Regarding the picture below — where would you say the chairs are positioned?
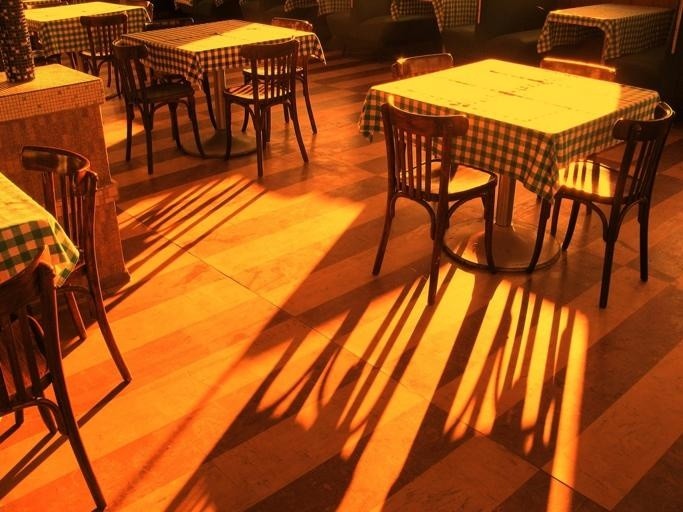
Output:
[0,141,130,433]
